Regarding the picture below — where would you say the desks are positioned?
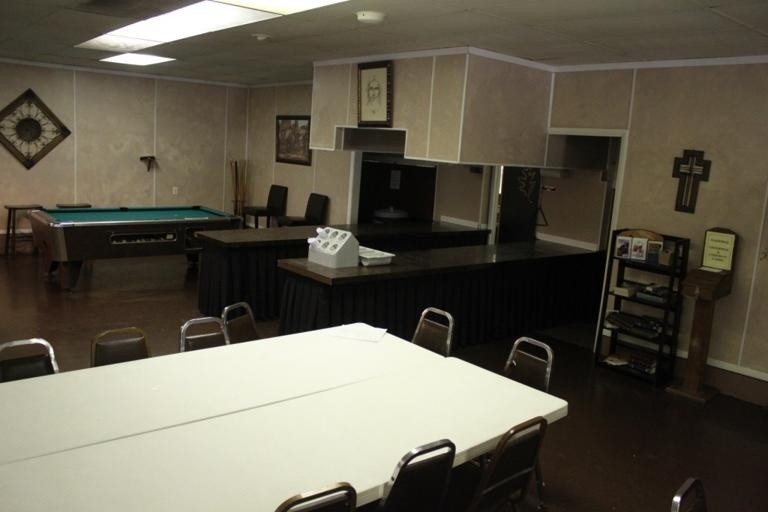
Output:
[28,206,242,290]
[195,219,492,322]
[276,240,596,334]
[0,321,569,512]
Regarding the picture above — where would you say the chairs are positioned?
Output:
[277,415,552,512]
[241,185,288,228]
[88,326,150,367]
[222,302,266,344]
[671,475,709,512]
[501,336,553,393]
[179,316,229,352]
[0,337,59,384]
[411,307,455,357]
[280,192,329,227]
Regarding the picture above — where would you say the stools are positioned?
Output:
[55,203,92,208]
[4,204,43,259]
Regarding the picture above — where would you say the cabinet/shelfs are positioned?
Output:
[585,228,691,400]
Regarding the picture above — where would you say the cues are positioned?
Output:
[229,162,246,214]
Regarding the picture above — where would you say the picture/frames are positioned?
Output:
[357,59,393,128]
[275,114,312,166]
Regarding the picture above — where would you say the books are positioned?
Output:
[630,237,649,261]
[614,236,632,260]
[614,282,677,304]
[629,356,657,374]
[646,241,664,262]
